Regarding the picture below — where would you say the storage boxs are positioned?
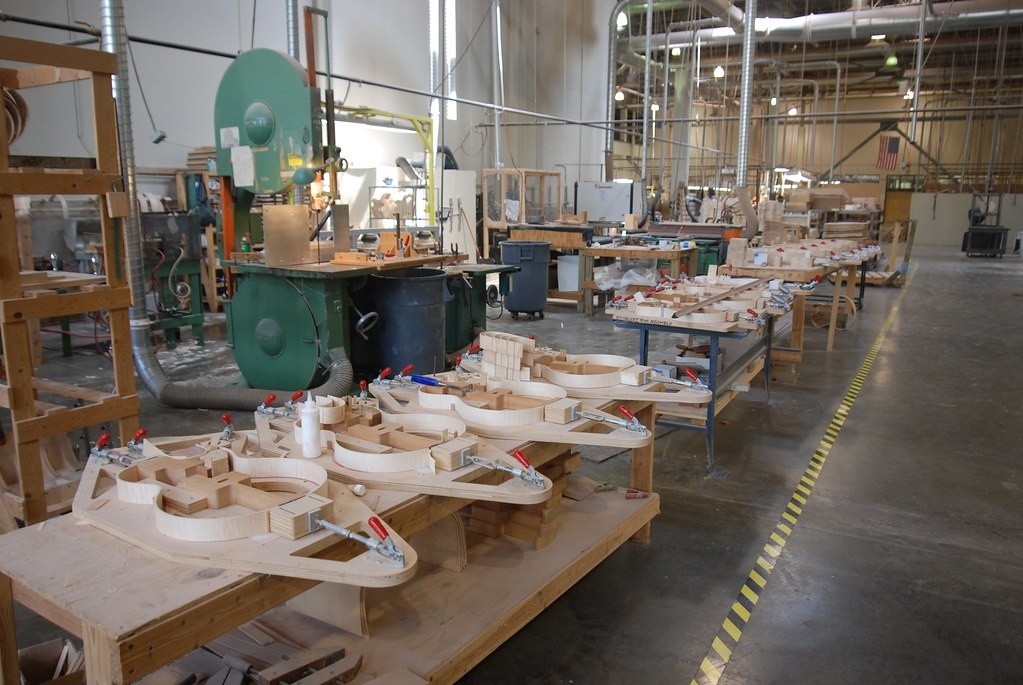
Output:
[676,350,722,373]
[654,364,679,379]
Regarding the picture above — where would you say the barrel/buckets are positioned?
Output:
[499,241,552,312]
[371,268,446,377]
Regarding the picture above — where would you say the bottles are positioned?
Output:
[301,390,322,458]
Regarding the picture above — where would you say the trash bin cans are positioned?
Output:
[367,269,452,381]
[499,240,552,321]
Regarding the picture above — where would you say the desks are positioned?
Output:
[1,362,665,684]
[582,246,699,318]
[718,242,884,379]
[605,286,799,471]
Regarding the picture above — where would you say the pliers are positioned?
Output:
[625,488,650,499]
[595,481,618,491]
